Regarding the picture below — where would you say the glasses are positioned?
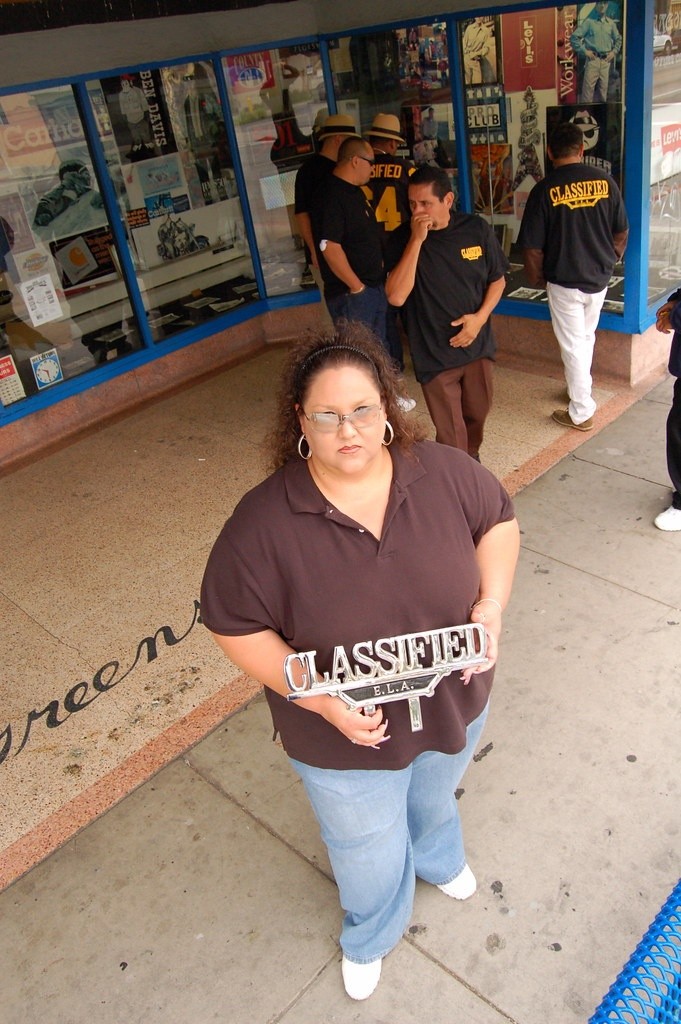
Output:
[301,403,385,433]
[357,156,375,166]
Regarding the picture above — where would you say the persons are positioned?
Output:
[655,288,681,531]
[463,18,492,84]
[570,1,622,104]
[294,113,417,412]
[31,160,132,366]
[516,123,629,432]
[201,322,519,1001]
[384,167,511,464]
[421,108,438,141]
[259,61,308,135]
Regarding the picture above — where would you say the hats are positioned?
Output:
[363,112,406,144]
[318,114,361,141]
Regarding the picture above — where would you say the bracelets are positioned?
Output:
[350,283,365,293]
[471,598,503,612]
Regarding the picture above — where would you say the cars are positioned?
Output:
[669,30,681,51]
[653,28,673,57]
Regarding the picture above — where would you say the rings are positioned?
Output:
[350,738,358,744]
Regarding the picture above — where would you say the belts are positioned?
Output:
[594,53,607,59]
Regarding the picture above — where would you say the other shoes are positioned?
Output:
[396,396,416,412]
[395,370,405,381]
[436,863,477,901]
[654,506,681,531]
[342,952,382,1000]
[553,408,593,430]
